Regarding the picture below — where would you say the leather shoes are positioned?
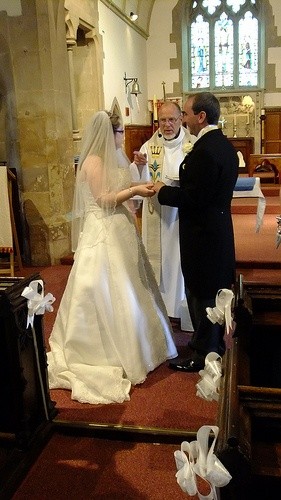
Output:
[169,356,205,371]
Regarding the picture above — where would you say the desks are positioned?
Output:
[220,274,281,500]
[249,153,281,179]
[0,272,50,500]
[225,136,254,174]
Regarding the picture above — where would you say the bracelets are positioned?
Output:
[129,188,134,198]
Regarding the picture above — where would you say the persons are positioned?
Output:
[129,102,196,333]
[45,109,179,405]
[148,92,240,374]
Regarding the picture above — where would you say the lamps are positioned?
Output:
[130,12,139,21]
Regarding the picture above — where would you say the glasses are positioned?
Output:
[158,115,182,125]
[114,129,124,135]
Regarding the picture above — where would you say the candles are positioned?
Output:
[233,113,236,125]
[247,110,250,124]
